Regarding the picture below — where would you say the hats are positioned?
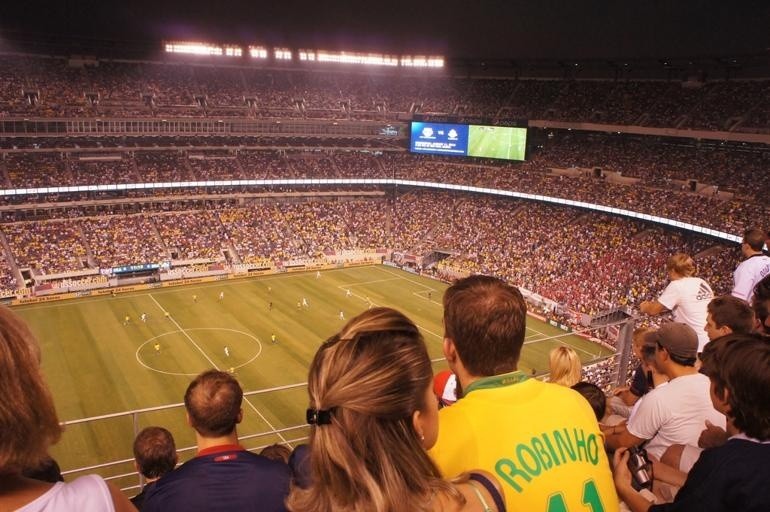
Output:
[644,322,698,359]
[432,371,457,406]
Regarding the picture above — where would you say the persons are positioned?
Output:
[0,47,769,512]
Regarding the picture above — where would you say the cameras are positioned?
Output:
[620,444,653,488]
